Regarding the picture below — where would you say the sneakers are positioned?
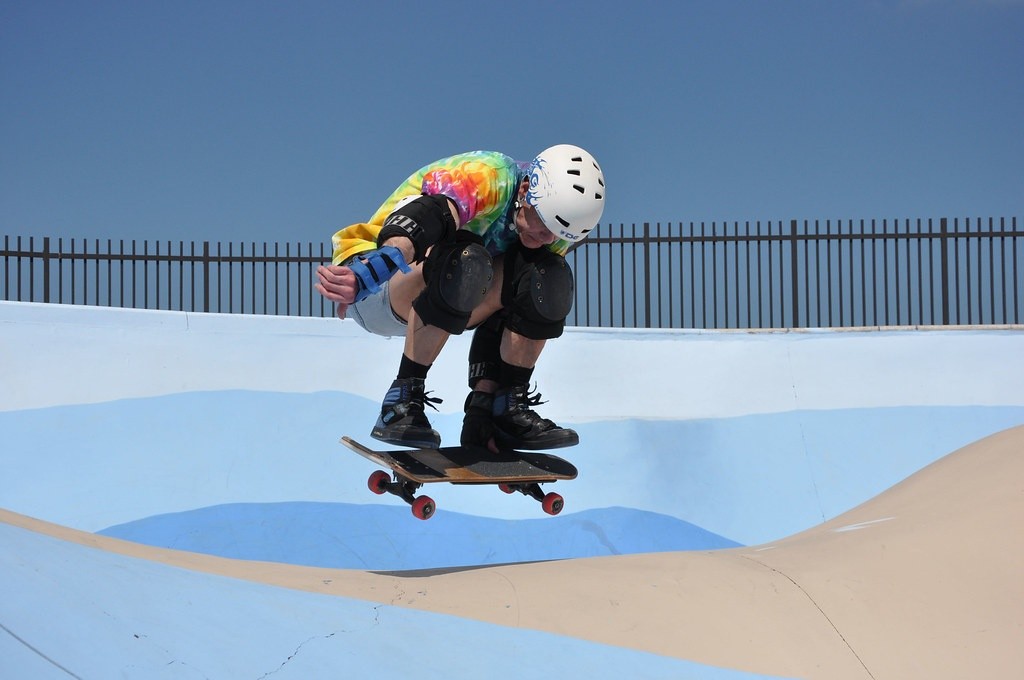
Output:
[370,378,443,451]
[461,391,580,451]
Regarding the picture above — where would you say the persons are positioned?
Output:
[315,144,605,453]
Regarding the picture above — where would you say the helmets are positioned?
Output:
[526,143,606,242]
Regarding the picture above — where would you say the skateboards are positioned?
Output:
[339,434,580,521]
